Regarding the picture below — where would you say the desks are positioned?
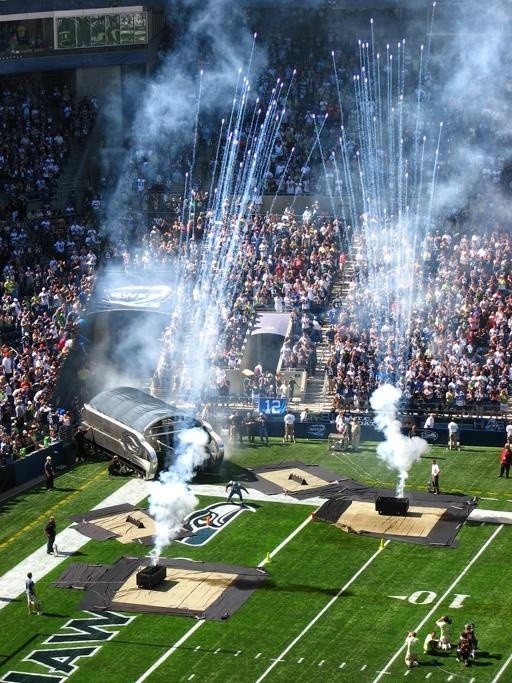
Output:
[327,433,351,452]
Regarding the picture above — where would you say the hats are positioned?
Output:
[46,456,52,460]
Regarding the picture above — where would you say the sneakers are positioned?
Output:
[424,651,430,655]
[444,642,475,667]
[410,661,421,667]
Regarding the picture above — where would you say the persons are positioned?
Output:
[403,631,419,667]
[498,442,512,478]
[45,456,58,491]
[422,630,442,654]
[434,615,453,650]
[455,632,471,666]
[0,1,511,468]
[43,515,57,555]
[106,454,122,476]
[427,458,441,494]
[462,623,477,660]
[25,572,44,615]
[224,480,250,503]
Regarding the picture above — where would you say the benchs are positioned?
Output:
[408,429,438,446]
